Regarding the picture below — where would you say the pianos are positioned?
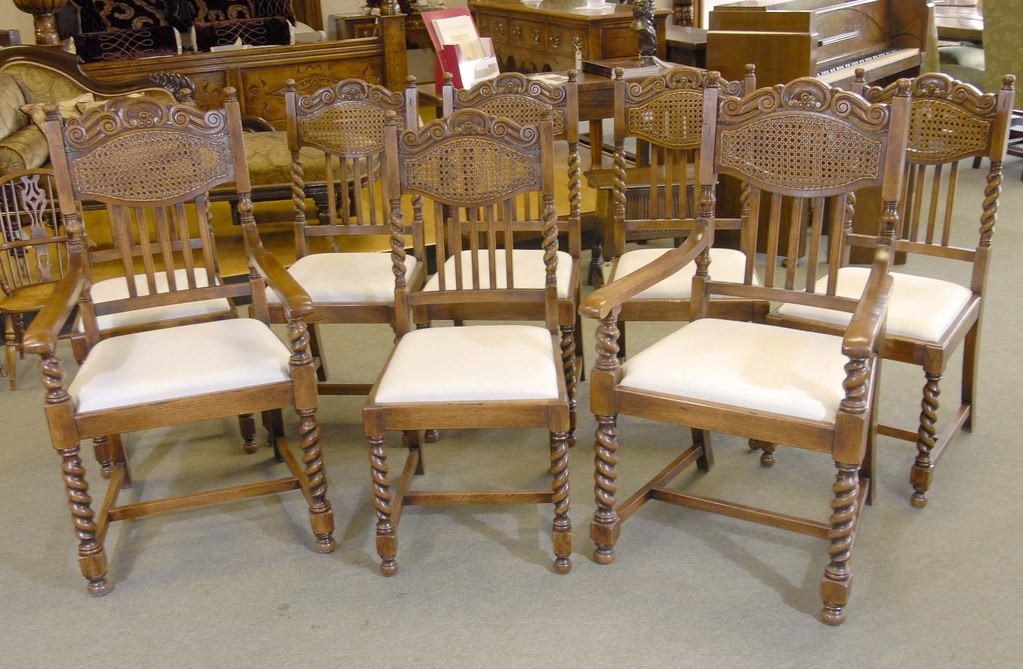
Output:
[705,0,935,266]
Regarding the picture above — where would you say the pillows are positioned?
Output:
[75,91,147,115]
[18,92,98,143]
[0,125,51,184]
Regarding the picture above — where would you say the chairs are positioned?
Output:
[242,78,434,452]
[753,69,1017,508]
[577,69,915,630]
[362,101,580,574]
[71,186,257,479]
[21,86,336,598]
[594,63,775,451]
[412,68,584,449]
[0,168,82,391]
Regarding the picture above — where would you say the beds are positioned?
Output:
[0,53,381,231]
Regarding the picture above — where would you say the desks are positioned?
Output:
[465,1,674,77]
[331,9,439,51]
